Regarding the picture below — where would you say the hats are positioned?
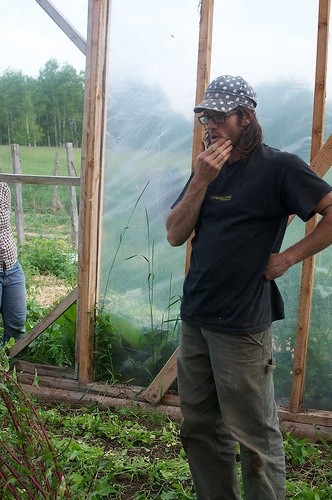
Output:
[193,75,258,114]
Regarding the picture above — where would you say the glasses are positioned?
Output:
[198,112,239,125]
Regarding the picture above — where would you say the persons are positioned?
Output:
[166,74,332,500]
[1,182,27,358]
